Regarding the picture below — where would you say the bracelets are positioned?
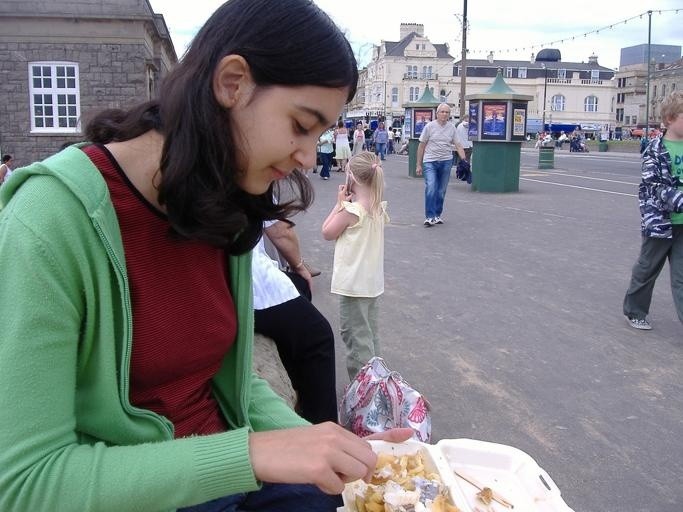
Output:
[295,258,307,271]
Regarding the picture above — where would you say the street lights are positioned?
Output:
[539,63,548,133]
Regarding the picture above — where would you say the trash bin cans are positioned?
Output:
[539,145,554,168]
[409,137,424,178]
[597,139,608,152]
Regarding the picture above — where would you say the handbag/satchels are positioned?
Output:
[337,356,435,445]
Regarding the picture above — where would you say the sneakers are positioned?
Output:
[623,311,652,331]
[285,257,322,277]
[423,218,435,226]
[323,158,386,181]
[434,216,444,224]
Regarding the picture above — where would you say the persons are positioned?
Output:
[619,90,682,331]
[415,102,473,229]
[313,113,400,180]
[0,3,428,512]
[0,153,13,184]
[532,129,665,153]
[240,195,335,434]
[317,148,389,394]
